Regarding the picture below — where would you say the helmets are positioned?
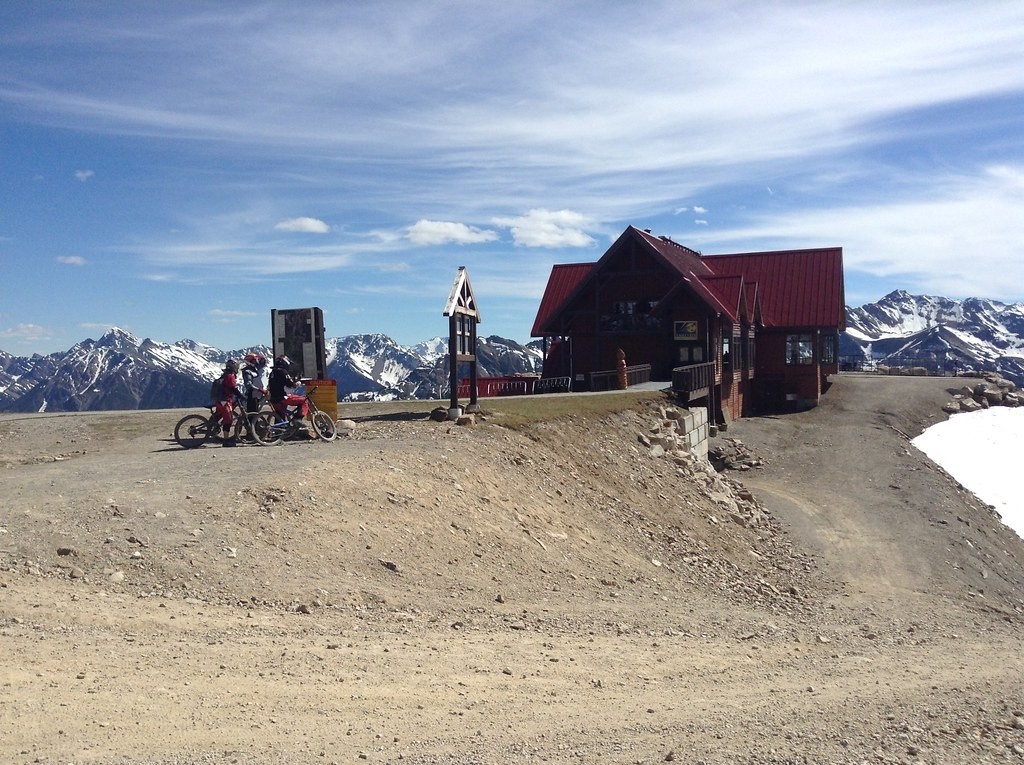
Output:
[225,358,240,374]
[275,355,295,367]
[243,354,259,368]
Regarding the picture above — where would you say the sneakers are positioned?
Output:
[295,420,308,428]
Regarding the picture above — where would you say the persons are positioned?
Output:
[209,359,247,447]
[268,356,308,440]
[241,353,269,440]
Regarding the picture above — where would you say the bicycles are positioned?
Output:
[250,385,336,447]
[235,388,301,445]
[174,386,269,448]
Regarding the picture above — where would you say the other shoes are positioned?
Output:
[222,442,237,447]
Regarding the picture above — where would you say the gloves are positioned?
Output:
[259,388,269,394]
[295,380,302,387]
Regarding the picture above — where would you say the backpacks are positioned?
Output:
[210,374,227,401]
